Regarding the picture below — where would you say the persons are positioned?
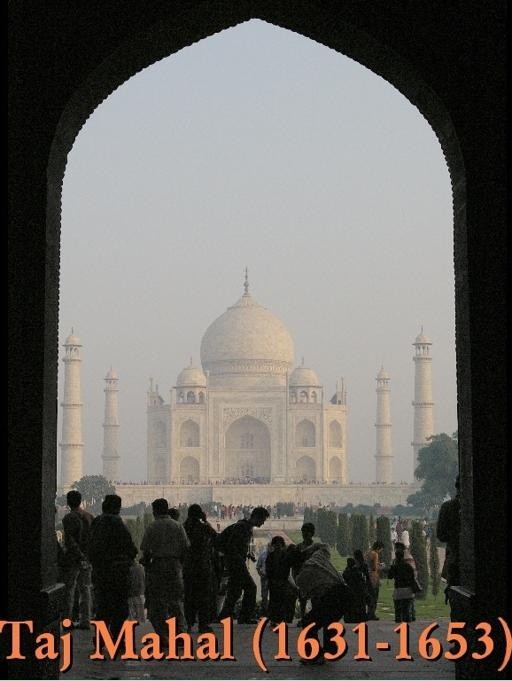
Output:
[54,490,433,665]
[436,474,460,657]
